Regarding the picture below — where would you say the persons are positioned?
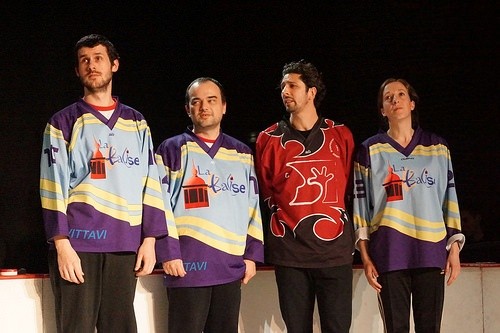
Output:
[354,79,465,333]
[154,77,264,333]
[255,63,354,333]
[40,33,169,333]
[460,206,500,263]
